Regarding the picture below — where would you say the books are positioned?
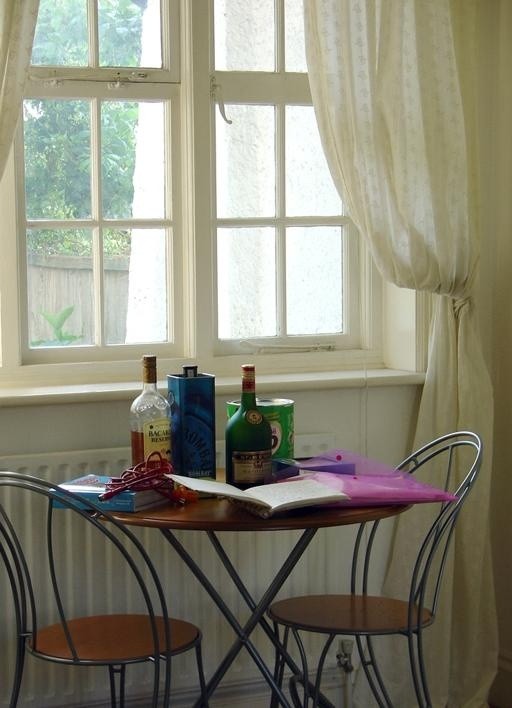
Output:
[48,473,171,513]
[163,471,352,519]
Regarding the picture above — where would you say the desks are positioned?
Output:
[90,466,412,707]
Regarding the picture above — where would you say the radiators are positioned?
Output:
[1,433,354,707]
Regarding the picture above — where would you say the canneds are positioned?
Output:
[226,398,293,462]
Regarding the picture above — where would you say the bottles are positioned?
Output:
[128,355,173,472]
[225,364,271,491]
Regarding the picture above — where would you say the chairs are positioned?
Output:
[0,470,207,708]
[269,431,483,707]
